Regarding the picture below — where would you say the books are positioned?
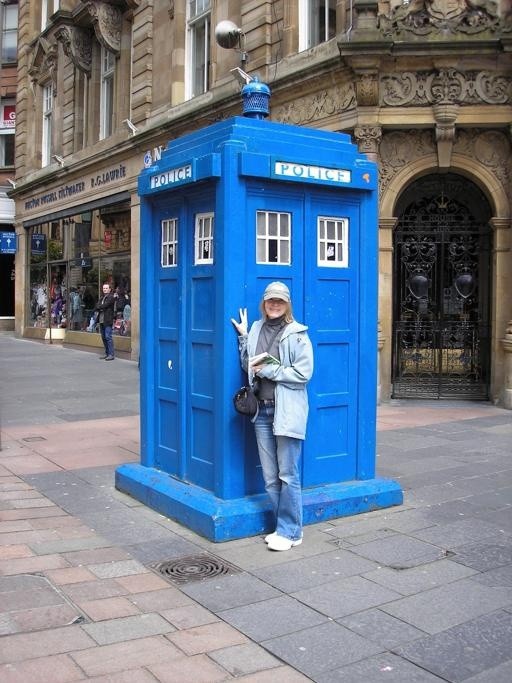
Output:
[249,351,280,366]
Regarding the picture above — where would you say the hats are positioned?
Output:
[264,281,290,303]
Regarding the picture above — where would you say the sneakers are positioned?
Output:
[99,355,114,360]
[264,530,304,551]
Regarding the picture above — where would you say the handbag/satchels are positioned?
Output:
[233,386,257,416]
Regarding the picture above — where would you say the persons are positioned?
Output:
[229,279,316,552]
[94,281,118,360]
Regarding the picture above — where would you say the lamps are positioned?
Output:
[214,19,248,60]
